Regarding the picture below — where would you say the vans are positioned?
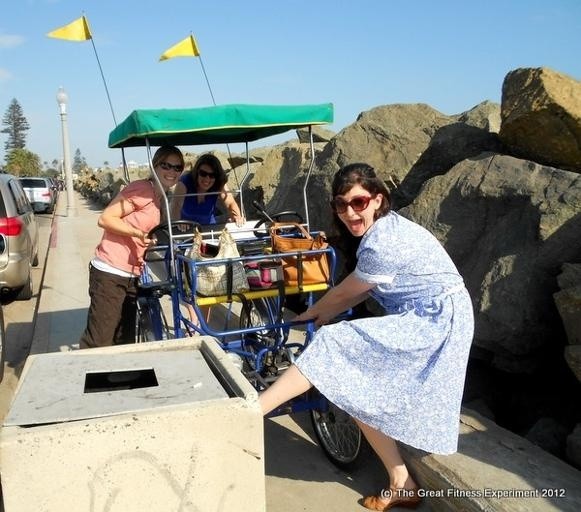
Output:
[0,173,40,302]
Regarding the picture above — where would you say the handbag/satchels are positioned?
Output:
[270,223,330,286]
[184,225,250,295]
[244,259,285,291]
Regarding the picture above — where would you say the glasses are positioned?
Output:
[158,161,182,172]
[198,168,217,179]
[329,195,376,214]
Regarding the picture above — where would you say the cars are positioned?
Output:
[17,175,57,214]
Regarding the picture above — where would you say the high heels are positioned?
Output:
[362,488,422,511]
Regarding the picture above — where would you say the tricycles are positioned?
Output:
[105,100,369,469]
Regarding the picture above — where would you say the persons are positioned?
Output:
[171,153,248,232]
[78,146,183,348]
[256,162,477,512]
